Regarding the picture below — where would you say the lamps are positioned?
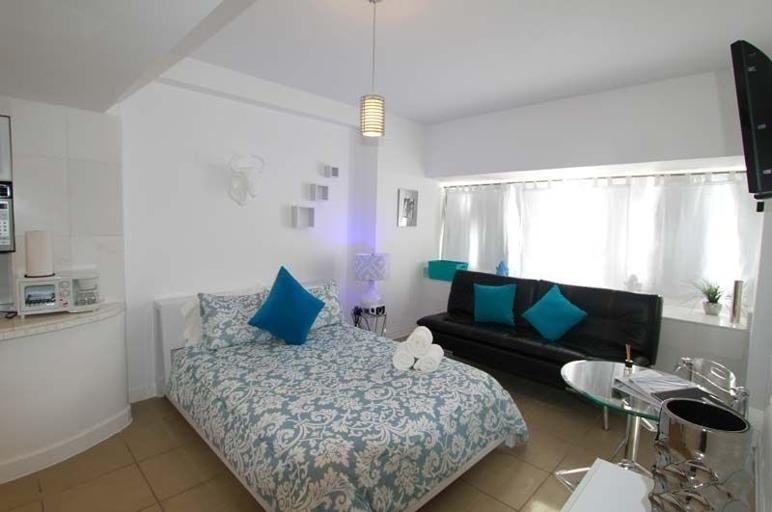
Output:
[353,251,390,306]
[361,0,385,136]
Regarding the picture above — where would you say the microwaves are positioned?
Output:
[16,270,73,320]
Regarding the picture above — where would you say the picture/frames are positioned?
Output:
[398,189,418,228]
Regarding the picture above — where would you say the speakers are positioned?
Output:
[370,303,386,316]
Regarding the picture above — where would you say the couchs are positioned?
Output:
[417,269,663,431]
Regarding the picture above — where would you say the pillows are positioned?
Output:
[198,266,345,350]
[473,283,517,325]
[521,284,588,343]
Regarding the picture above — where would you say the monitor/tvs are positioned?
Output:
[730,37,772,212]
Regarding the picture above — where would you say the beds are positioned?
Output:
[154,285,529,512]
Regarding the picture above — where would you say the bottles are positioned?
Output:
[623,358,634,376]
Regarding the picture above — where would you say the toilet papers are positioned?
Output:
[24,229,54,277]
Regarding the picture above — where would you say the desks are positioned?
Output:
[561,359,719,479]
[554,455,654,511]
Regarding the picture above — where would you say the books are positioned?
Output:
[651,386,725,406]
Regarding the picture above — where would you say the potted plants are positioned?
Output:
[679,278,733,316]
